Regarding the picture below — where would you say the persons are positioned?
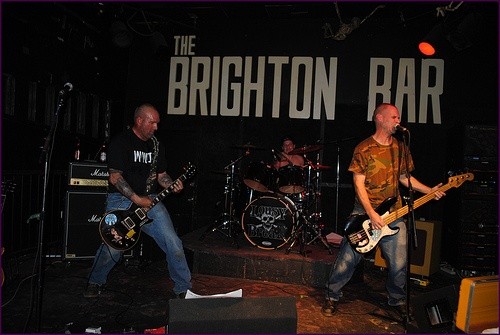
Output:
[322,103,446,324]
[84,104,193,300]
[275,138,302,187]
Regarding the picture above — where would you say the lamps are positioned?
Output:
[418,37,440,56]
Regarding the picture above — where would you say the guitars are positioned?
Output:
[99,162,197,250]
[344,173,474,254]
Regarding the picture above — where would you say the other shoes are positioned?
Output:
[179,292,187,299]
[396,303,408,316]
[321,299,336,317]
[84,283,99,297]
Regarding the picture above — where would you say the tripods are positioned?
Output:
[203,149,340,257]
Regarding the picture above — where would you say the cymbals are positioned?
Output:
[230,145,267,152]
[289,145,322,155]
[301,164,334,168]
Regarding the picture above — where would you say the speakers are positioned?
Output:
[64,191,111,260]
[374,220,441,276]
[412,283,460,335]
[167,296,298,335]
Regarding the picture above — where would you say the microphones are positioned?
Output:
[59,83,73,95]
[394,123,408,132]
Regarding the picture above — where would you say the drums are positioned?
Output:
[276,164,303,193]
[242,193,300,250]
[242,161,278,192]
